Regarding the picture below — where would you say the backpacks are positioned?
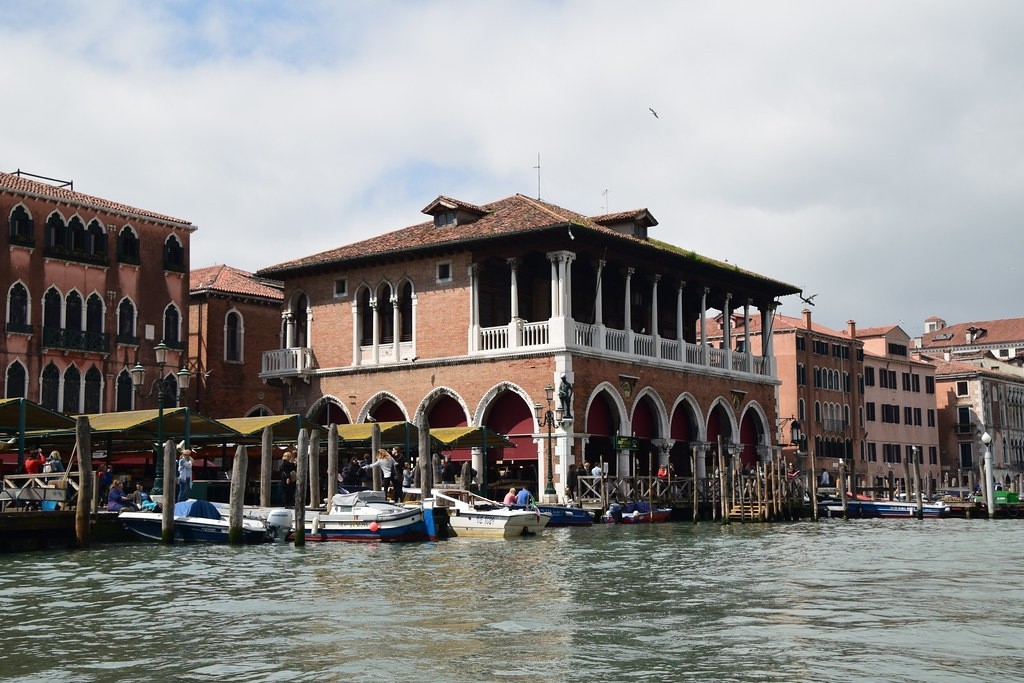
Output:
[42,459,56,473]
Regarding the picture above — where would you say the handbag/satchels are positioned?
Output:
[14,463,25,488]
[281,475,291,487]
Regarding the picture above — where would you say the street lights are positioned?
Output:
[534,383,564,494]
[130,340,192,494]
[982,432,995,514]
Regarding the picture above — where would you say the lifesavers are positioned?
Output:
[1006,504,1020,518]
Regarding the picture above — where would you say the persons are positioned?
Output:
[441,455,456,483]
[504,486,531,505]
[558,374,573,415]
[821,468,830,487]
[98,464,113,508]
[659,463,675,495]
[134,482,158,512]
[402,456,421,501]
[346,453,373,486]
[26,448,46,474]
[44,450,65,482]
[177,450,193,502]
[108,479,138,512]
[788,462,800,477]
[280,450,297,508]
[742,464,756,475]
[567,462,601,502]
[362,445,405,503]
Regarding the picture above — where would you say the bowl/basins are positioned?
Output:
[41,501,58,511]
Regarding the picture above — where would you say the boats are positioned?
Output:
[286,491,423,542]
[117,491,292,544]
[602,503,672,524]
[802,482,1024,518]
[537,505,596,527]
[398,488,553,538]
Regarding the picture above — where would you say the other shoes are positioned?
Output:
[285,505,293,509]
